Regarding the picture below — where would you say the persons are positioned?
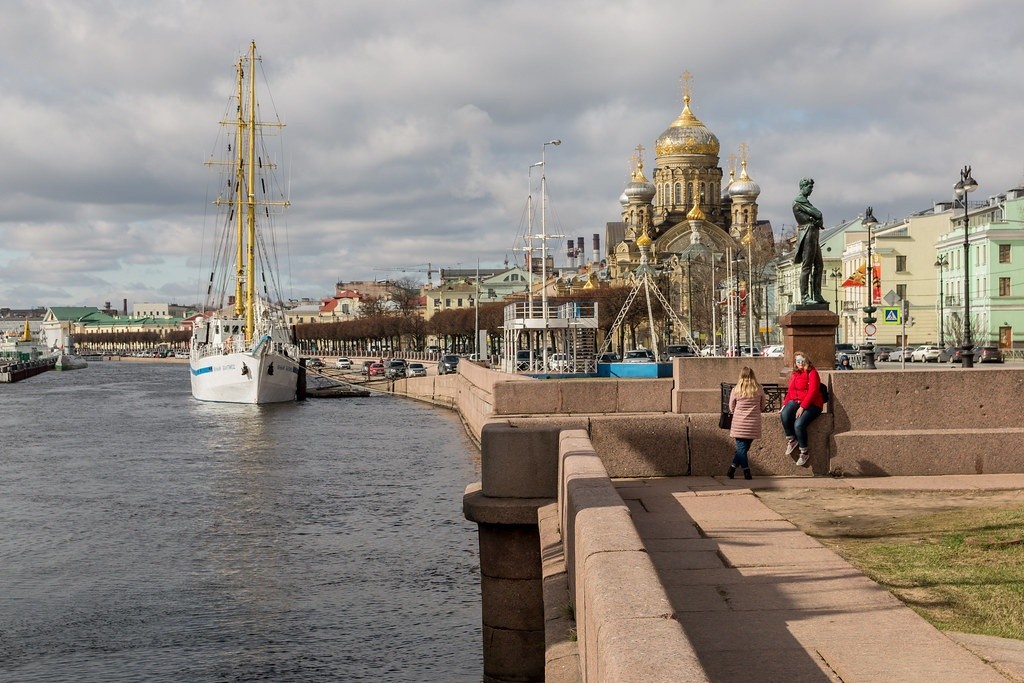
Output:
[792,176,830,305]
[836,355,854,370]
[729,366,766,479]
[779,351,823,466]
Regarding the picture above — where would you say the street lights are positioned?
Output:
[526,139,562,372]
[952,164,978,369]
[829,266,843,344]
[860,206,878,369]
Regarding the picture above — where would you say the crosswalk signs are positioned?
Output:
[885,310,898,321]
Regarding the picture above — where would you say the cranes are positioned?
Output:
[373,261,440,283]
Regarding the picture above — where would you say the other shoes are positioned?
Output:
[727,466,736,479]
[785,440,798,455]
[796,454,809,466]
[744,469,752,479]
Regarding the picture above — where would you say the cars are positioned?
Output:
[119,350,190,359]
[336,358,351,370]
[423,346,440,354]
[437,355,462,375]
[361,360,384,376]
[836,343,1004,364]
[517,349,541,369]
[89,350,114,356]
[302,357,322,366]
[549,353,574,370]
[595,343,786,363]
[385,358,428,379]
[469,354,490,363]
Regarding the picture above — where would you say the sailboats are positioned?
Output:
[188,39,306,404]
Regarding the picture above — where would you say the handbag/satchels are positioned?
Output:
[820,382,828,404]
[719,411,733,429]
